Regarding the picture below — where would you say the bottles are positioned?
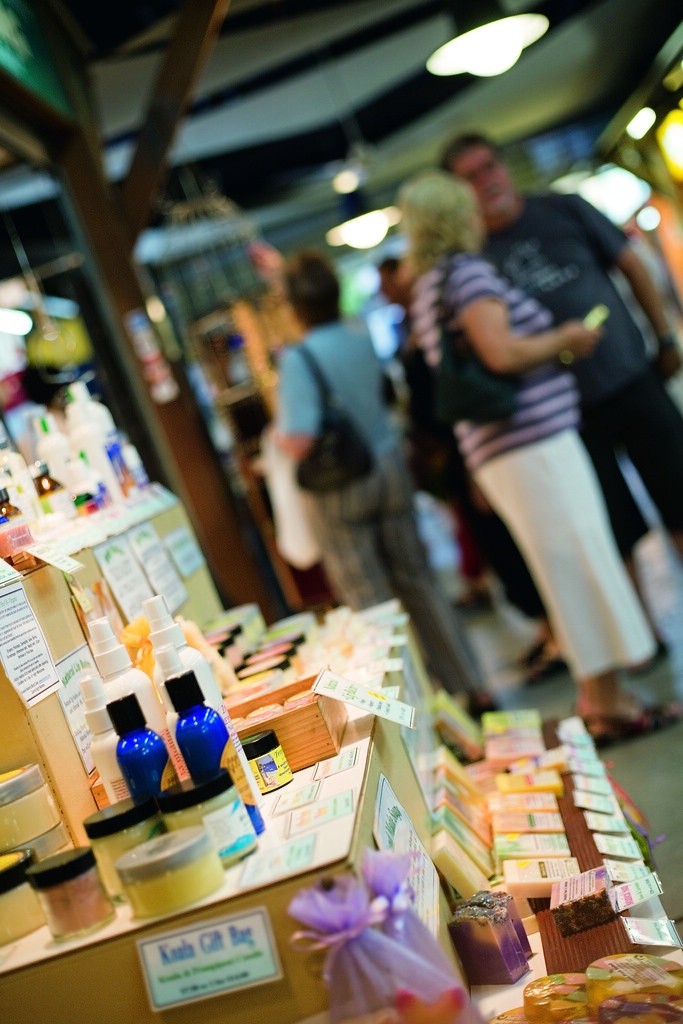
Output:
[142,594,265,810]
[153,643,182,757]
[106,692,180,799]
[165,669,266,835]
[0,381,147,556]
[87,616,191,783]
[76,674,132,805]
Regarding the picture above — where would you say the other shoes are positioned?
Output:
[593,700,681,750]
[466,690,498,721]
[523,641,545,666]
[453,590,491,610]
[631,641,667,674]
[526,658,566,684]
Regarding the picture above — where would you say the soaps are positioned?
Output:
[447,890,533,984]
[550,866,618,937]
[429,688,582,902]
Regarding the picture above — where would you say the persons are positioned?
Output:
[399,171,682,747]
[440,132,683,679]
[377,257,567,691]
[274,250,502,722]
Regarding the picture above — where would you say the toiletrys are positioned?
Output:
[0,380,149,558]
[142,595,266,813]
[165,671,266,836]
[106,693,180,798]
[87,616,191,783]
[80,674,131,805]
[154,644,218,778]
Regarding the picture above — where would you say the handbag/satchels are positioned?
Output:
[260,432,322,569]
[432,256,518,423]
[295,345,377,492]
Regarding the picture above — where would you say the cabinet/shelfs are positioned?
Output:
[0,502,683,1023]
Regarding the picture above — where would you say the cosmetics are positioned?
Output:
[1,382,370,937]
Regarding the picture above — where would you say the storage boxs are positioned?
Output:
[228,675,349,772]
